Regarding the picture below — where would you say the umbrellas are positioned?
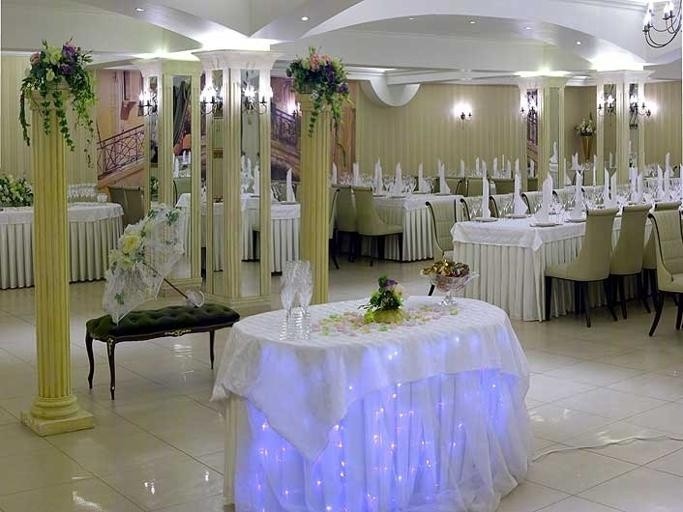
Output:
[101,208,206,322]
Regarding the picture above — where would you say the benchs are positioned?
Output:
[85,303,240,400]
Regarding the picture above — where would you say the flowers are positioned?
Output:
[281,44,356,168]
[0,169,34,210]
[18,36,98,168]
[574,117,598,137]
[356,273,408,325]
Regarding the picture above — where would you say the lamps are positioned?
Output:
[595,91,654,129]
[196,85,224,118]
[240,83,274,115]
[642,0,681,48]
[137,90,158,117]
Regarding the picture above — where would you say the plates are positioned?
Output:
[529,221,563,228]
[436,193,450,196]
[565,217,586,223]
[373,194,386,197]
[413,191,427,195]
[250,194,260,198]
[281,200,297,205]
[505,214,527,218]
[472,216,499,222]
[391,194,406,198]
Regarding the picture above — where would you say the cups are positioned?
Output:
[281,260,296,325]
[297,260,311,325]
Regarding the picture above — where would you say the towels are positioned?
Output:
[610,152,613,168]
[183,151,186,162]
[571,155,578,170]
[506,160,511,179]
[679,164,683,199]
[173,152,175,168]
[631,167,636,205]
[419,163,423,190]
[286,167,295,201]
[460,159,465,177]
[254,165,259,195]
[241,154,245,171]
[665,152,674,177]
[661,170,670,203]
[438,158,441,174]
[482,160,487,178]
[482,177,491,219]
[531,174,552,222]
[247,157,251,177]
[529,159,535,179]
[611,171,617,210]
[551,142,558,163]
[439,163,450,193]
[332,161,337,184]
[514,174,528,215]
[574,152,578,165]
[515,157,520,175]
[188,151,191,164]
[493,156,497,175]
[353,162,360,185]
[593,154,597,169]
[476,157,479,175]
[174,158,180,178]
[604,168,611,209]
[375,159,383,195]
[628,140,632,156]
[570,170,584,219]
[637,171,644,204]
[395,162,402,196]
[658,166,662,198]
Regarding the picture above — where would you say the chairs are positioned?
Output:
[434,179,462,195]
[464,177,491,197]
[610,203,652,321]
[643,200,683,337]
[543,207,619,328]
[316,186,342,274]
[352,186,406,269]
[424,181,683,298]
[491,178,538,194]
[336,186,360,263]
